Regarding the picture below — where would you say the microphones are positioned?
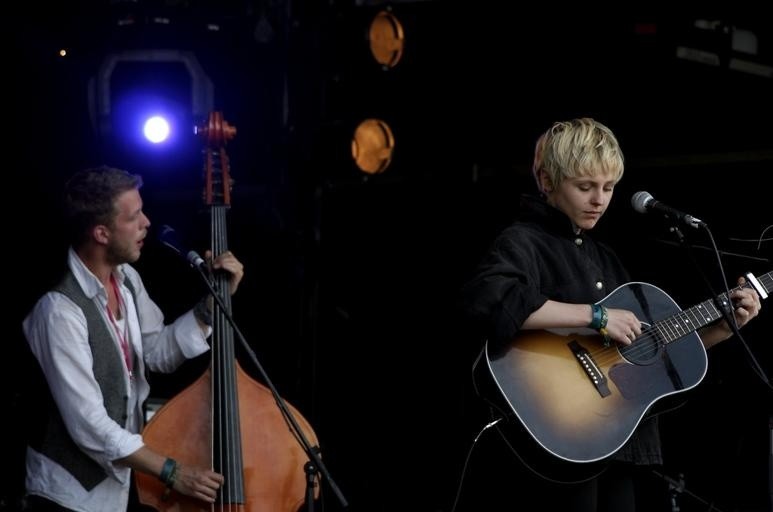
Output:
[154,223,205,267]
[631,189,704,230]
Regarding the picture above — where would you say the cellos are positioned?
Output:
[129,113,321,510]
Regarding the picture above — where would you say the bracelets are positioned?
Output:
[588,303,603,331]
[159,457,176,484]
[194,297,213,327]
[595,304,608,335]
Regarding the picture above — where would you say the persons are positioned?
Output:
[453,112,763,511]
[13,163,247,511]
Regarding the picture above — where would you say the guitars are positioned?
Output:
[470,270,773,485]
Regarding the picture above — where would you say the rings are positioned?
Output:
[628,331,634,338]
